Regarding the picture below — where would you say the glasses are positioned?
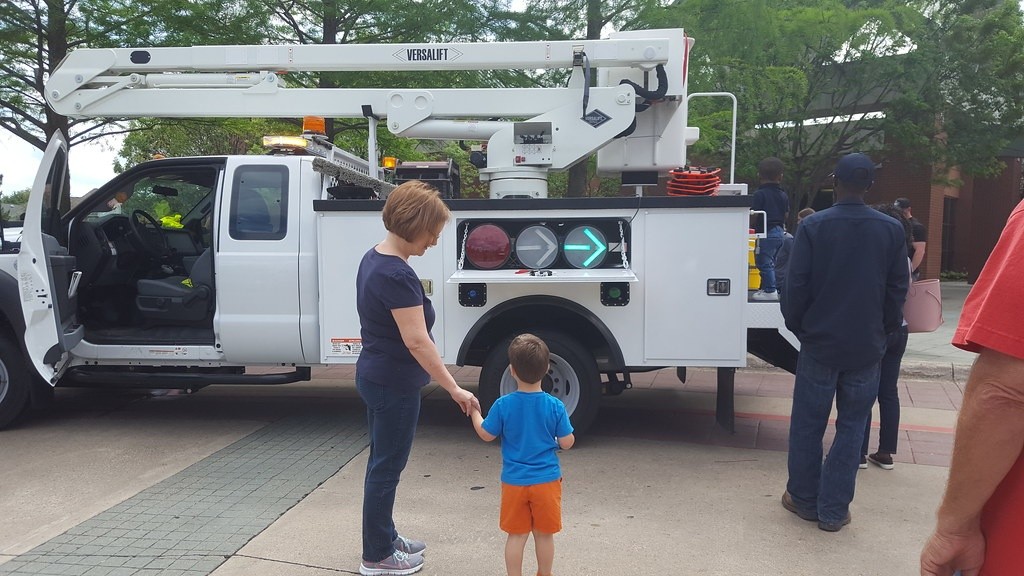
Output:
[427,228,440,242]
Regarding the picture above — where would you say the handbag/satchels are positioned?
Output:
[900,257,944,332]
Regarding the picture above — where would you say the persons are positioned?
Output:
[471,333,575,576]
[748,157,788,301]
[921,199,1024,576]
[796,208,815,226]
[782,154,909,531]
[858,198,927,469]
[356,181,482,576]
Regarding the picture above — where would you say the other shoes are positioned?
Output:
[782,490,818,520]
[817,510,851,531]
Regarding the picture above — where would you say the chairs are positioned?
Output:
[134,189,273,326]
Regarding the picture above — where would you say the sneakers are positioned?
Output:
[859,455,867,468]
[868,452,894,469]
[391,535,427,556]
[753,289,778,299]
[358,549,423,576]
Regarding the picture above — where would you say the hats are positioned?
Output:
[892,197,912,209]
[757,157,785,180]
[828,152,876,182]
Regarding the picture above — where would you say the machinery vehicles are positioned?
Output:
[0,28,801,430]
